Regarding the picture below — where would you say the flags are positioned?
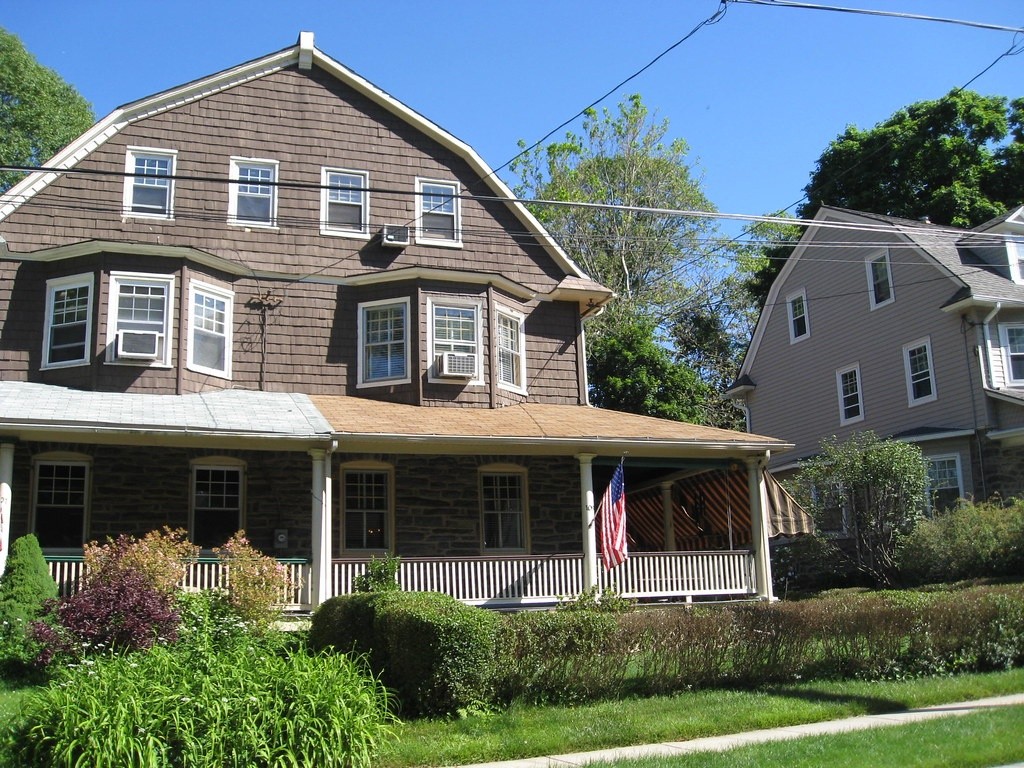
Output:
[600,457,628,571]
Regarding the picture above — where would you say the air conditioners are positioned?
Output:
[381,224,410,247]
[118,329,159,360]
[437,350,478,378]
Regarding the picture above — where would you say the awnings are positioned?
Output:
[625,465,814,553]
